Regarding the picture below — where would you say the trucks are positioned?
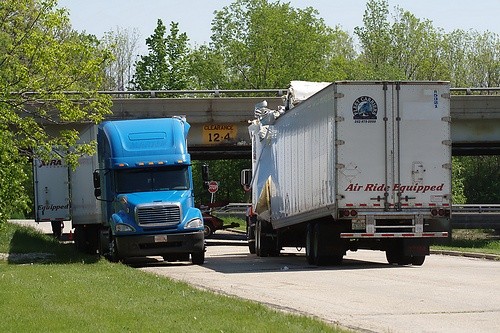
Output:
[32,116,205,266]
[240,81,453,264]
